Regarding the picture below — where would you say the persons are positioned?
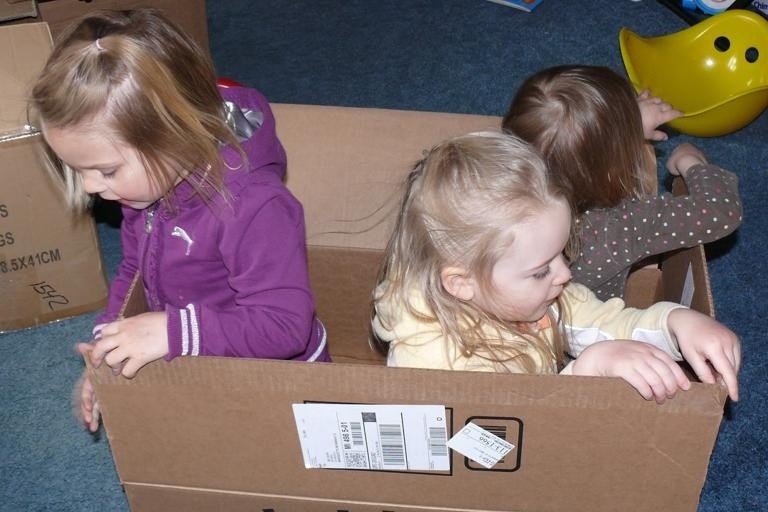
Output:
[367,130,742,408]
[26,7,334,433]
[498,64,742,304]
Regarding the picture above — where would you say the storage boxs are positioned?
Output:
[81,103,728,512]
